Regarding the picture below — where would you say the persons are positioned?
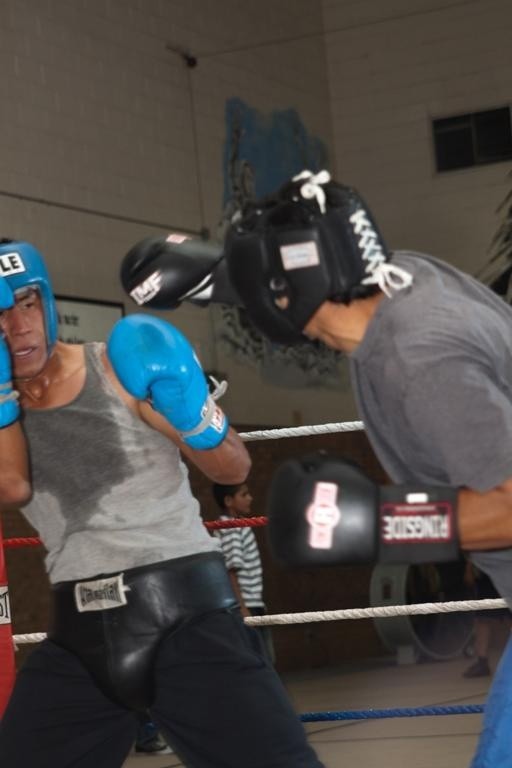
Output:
[213,481,276,666]
[121,173,512,768]
[462,564,507,679]
[136,721,173,754]
[0,235,324,768]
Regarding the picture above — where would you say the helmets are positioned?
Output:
[224,171,390,343]
[0,241,57,354]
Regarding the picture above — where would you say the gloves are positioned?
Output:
[120,235,224,308]
[0,274,21,428]
[270,451,378,570]
[108,313,228,450]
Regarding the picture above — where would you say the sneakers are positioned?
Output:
[136,741,173,754]
[463,662,489,676]
